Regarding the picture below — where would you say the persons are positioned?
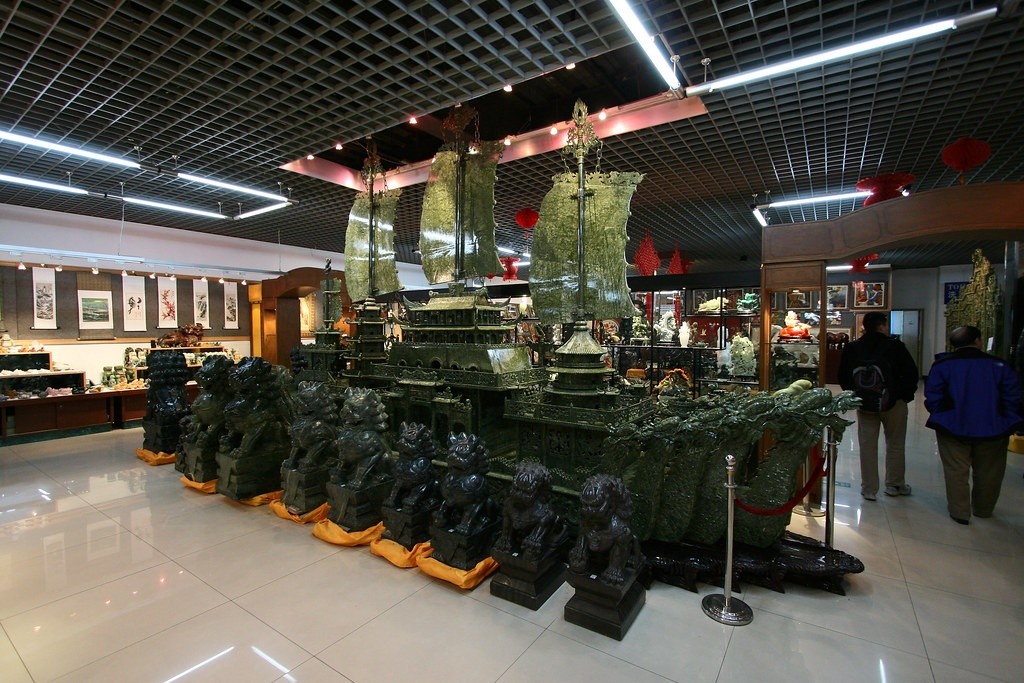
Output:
[838,312,919,502]
[923,325,1024,525]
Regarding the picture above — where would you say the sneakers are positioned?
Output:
[884,484,911,496]
[861,489,876,500]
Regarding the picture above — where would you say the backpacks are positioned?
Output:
[849,363,889,411]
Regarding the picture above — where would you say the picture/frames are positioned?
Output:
[723,289,745,312]
[749,286,778,311]
[852,280,886,309]
[692,289,716,311]
[818,285,848,310]
[30,265,240,341]
[783,291,812,310]
